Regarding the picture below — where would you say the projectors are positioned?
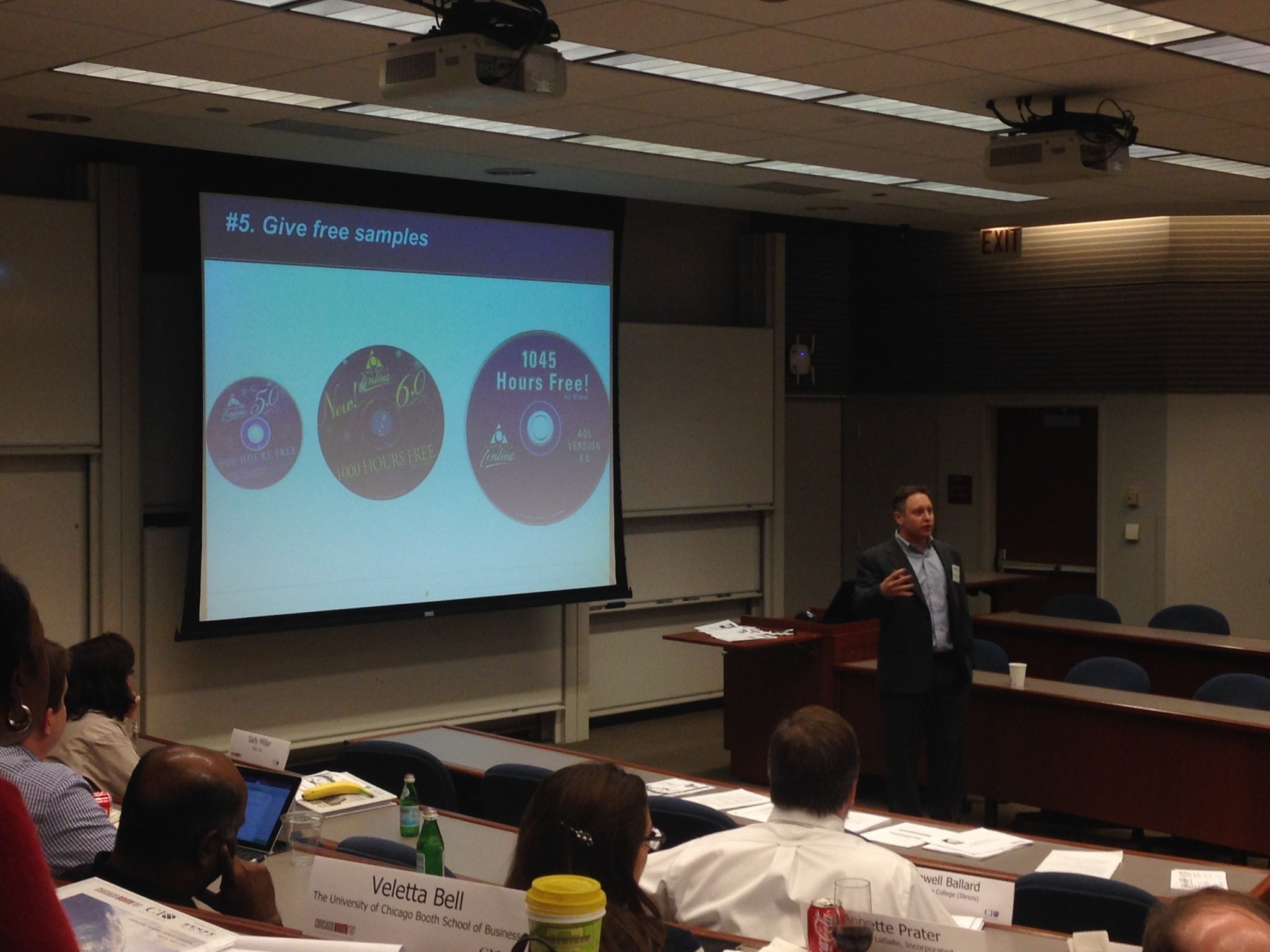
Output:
[377,31,570,104]
[983,128,1130,184]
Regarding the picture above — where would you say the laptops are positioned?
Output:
[235,763,304,866]
[815,578,854,624]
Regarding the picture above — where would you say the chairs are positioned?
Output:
[325,594,1270,952]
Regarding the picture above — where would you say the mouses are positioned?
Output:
[794,610,815,620]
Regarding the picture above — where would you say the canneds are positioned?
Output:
[94,791,113,818]
[807,898,845,952]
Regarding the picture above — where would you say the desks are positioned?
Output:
[957,607,1270,664]
[341,723,1270,932]
[113,771,1142,952]
[840,653,1270,861]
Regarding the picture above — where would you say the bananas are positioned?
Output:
[301,781,374,800]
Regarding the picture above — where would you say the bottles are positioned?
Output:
[399,773,421,839]
[415,806,445,878]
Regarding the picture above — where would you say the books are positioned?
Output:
[846,810,957,849]
[55,876,239,952]
[923,827,1036,861]
[952,914,985,931]
[295,770,397,819]
[646,776,775,825]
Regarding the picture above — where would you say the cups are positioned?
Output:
[523,875,607,952]
[279,811,326,867]
[830,878,874,952]
[1009,663,1027,687]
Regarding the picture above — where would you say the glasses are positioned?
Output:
[634,827,665,850]
[126,667,135,677]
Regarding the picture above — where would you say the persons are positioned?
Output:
[43,632,141,805]
[850,485,975,824]
[637,705,958,950]
[0,639,120,880]
[59,745,284,927]
[503,762,703,952]
[0,566,79,952]
